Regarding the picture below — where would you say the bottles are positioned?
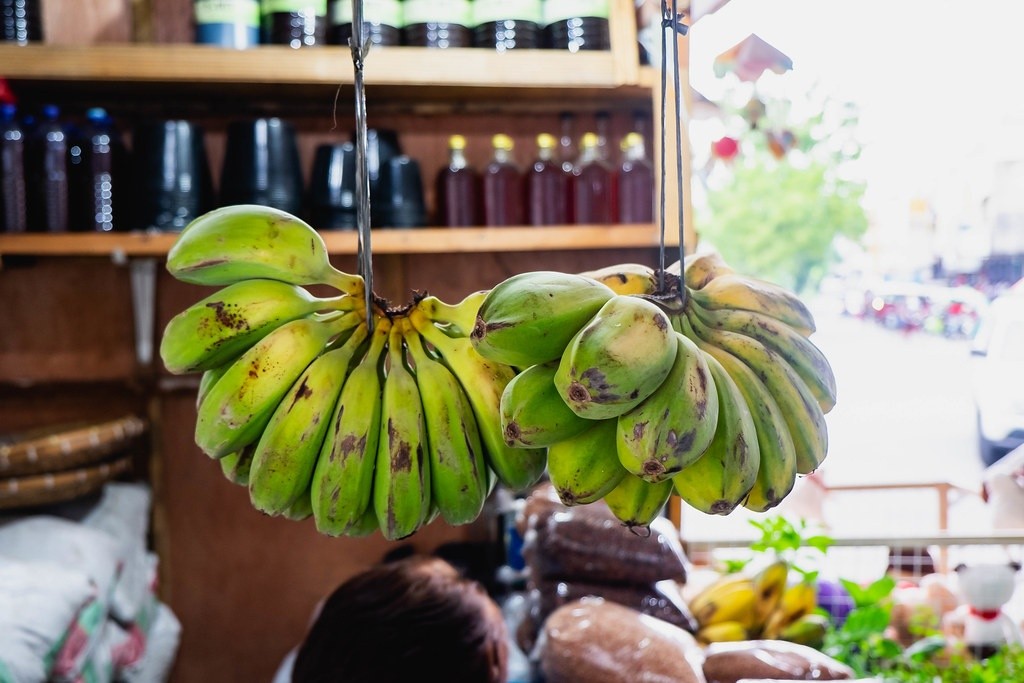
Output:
[437,134,480,226]
[324,1,404,47]
[400,0,475,46]
[140,120,209,228]
[26,103,129,235]
[351,129,405,228]
[376,156,427,228]
[219,116,304,218]
[544,0,607,52]
[572,131,611,227]
[259,1,328,47]
[309,142,360,228]
[479,133,528,228]
[472,0,542,49]
[595,110,621,225]
[613,132,654,226]
[524,131,568,225]
[0,101,35,233]
[1,0,43,48]
[557,107,578,225]
[632,112,653,158]
[193,0,259,45]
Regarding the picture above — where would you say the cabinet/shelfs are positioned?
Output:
[0,0,692,250]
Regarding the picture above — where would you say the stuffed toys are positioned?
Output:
[945,560,1021,651]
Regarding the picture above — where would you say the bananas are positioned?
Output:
[160,200,838,543]
